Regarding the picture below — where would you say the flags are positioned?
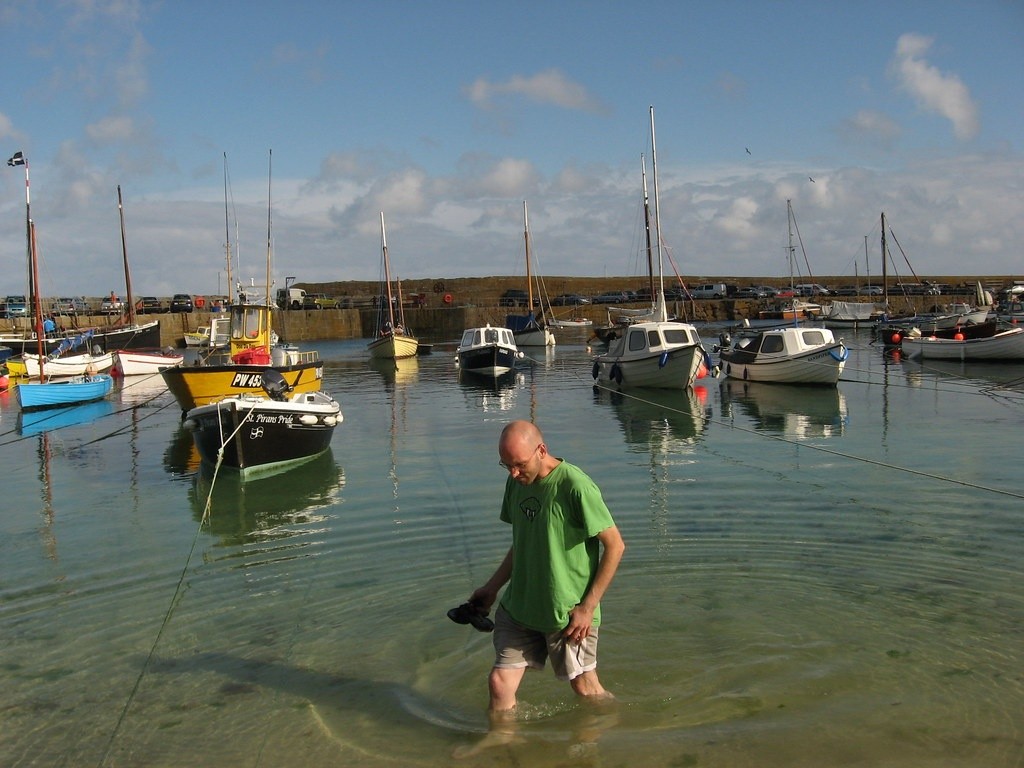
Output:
[6,149,27,167]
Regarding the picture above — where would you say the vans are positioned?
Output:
[795,283,830,297]
[275,289,308,310]
[689,283,727,300]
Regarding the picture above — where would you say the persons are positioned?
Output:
[43,312,56,332]
[381,321,403,335]
[448,421,625,763]
[107,290,120,318]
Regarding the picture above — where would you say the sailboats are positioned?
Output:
[14,400,113,517]
[493,200,556,346]
[366,356,420,458]
[367,211,434,360]
[0,184,163,349]
[158,148,329,416]
[587,107,712,390]
[12,221,114,410]
[22,158,116,378]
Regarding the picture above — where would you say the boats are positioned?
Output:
[714,306,848,386]
[859,213,1024,363]
[184,368,343,485]
[111,349,186,377]
[0,373,10,389]
[548,316,594,328]
[455,322,525,377]
[6,352,28,377]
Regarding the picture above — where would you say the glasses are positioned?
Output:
[499,444,541,470]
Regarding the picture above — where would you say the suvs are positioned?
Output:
[497,289,541,308]
[592,290,629,305]
[302,293,340,310]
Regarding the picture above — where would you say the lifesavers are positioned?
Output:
[193,295,206,308]
[444,293,453,303]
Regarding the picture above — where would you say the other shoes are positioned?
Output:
[447,602,495,633]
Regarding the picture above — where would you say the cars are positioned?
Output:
[550,292,592,306]
[167,294,193,314]
[4,295,29,318]
[859,285,884,297]
[733,287,767,300]
[101,297,123,316]
[837,285,858,295]
[777,287,802,297]
[635,287,679,301]
[757,285,781,297]
[49,297,93,316]
[136,297,162,315]
